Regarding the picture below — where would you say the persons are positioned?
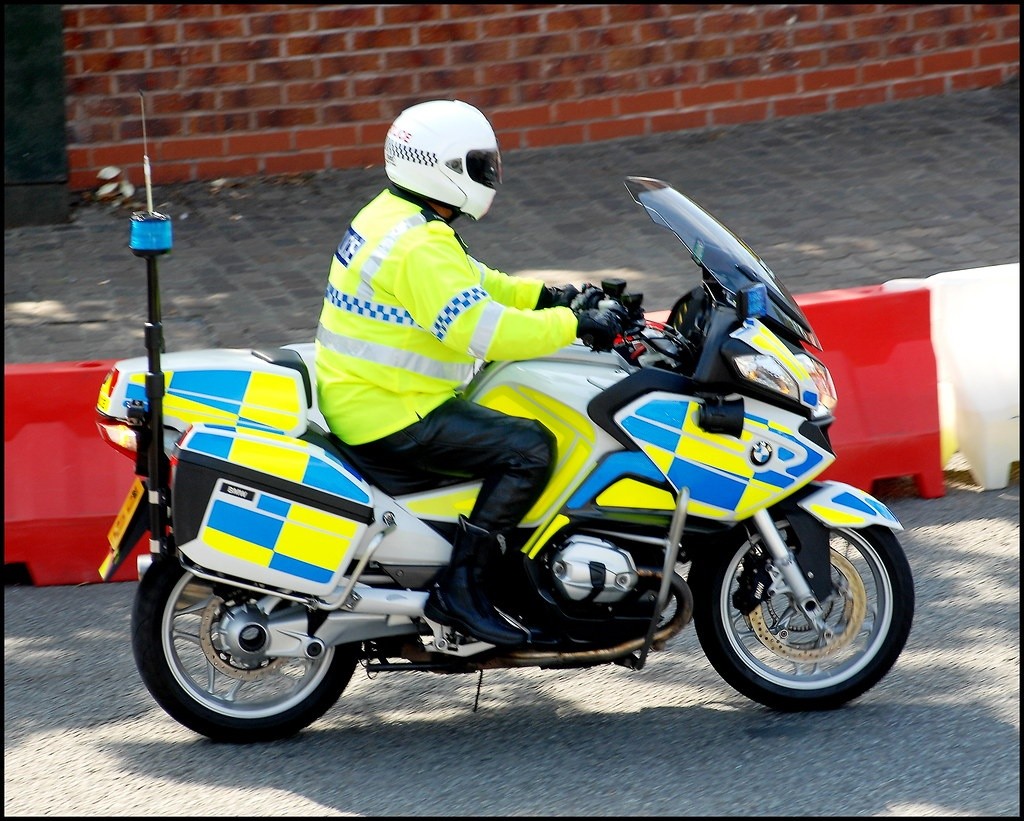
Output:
[314,99,620,645]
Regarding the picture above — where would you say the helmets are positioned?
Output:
[384,99,502,222]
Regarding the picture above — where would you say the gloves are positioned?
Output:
[537,282,596,309]
[576,308,620,354]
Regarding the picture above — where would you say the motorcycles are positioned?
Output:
[93,93,915,745]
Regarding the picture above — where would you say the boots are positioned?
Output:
[423,514,532,647]
